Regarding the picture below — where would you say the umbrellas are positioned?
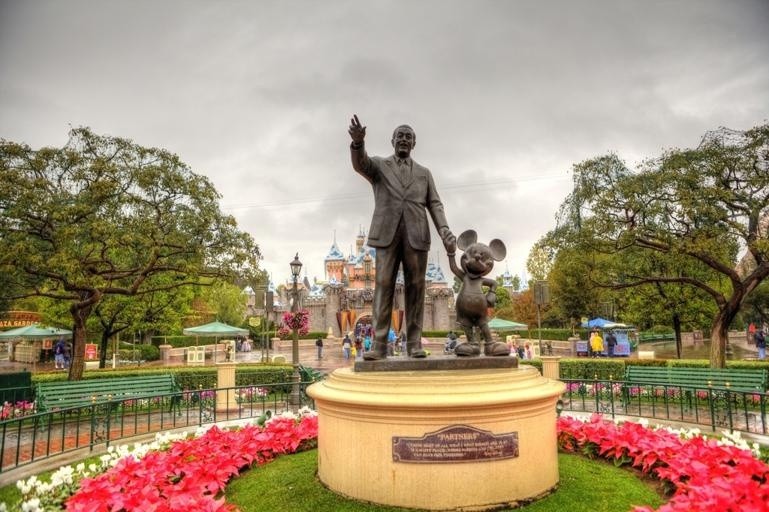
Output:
[183,321,249,363]
[581,317,611,333]
[487,316,529,342]
[0,322,74,376]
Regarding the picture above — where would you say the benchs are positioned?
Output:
[34,373,183,433]
[620,364,768,420]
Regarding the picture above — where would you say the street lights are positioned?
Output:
[290,251,303,410]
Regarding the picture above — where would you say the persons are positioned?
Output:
[754,325,767,359]
[505,337,532,360]
[54,338,73,369]
[445,330,457,351]
[349,113,448,361]
[315,326,407,359]
[238,334,251,351]
[587,329,618,358]
